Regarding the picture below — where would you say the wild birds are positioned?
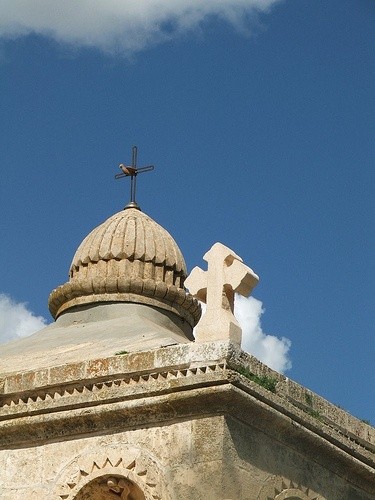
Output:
[119,164,137,176]
